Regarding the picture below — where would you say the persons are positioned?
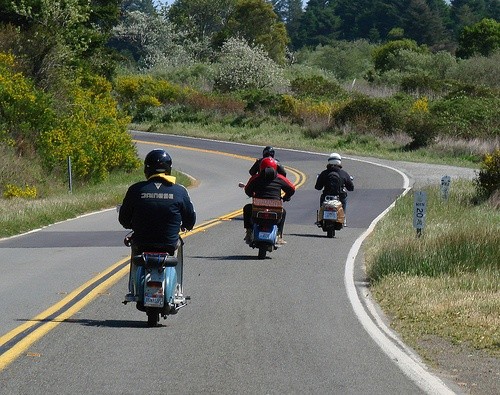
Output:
[118,150,196,303]
[249,145,287,178]
[243,157,295,245]
[314,153,354,227]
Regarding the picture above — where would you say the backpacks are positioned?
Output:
[325,171,341,195]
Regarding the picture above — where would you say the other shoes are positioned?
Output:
[275,239,287,244]
[245,236,253,243]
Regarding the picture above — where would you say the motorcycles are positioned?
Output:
[237,182,290,259]
[123,222,193,327]
[314,173,356,239]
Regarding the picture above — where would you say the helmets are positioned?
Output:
[144,149,173,177]
[263,146,274,158]
[326,153,341,166]
[260,158,277,173]
